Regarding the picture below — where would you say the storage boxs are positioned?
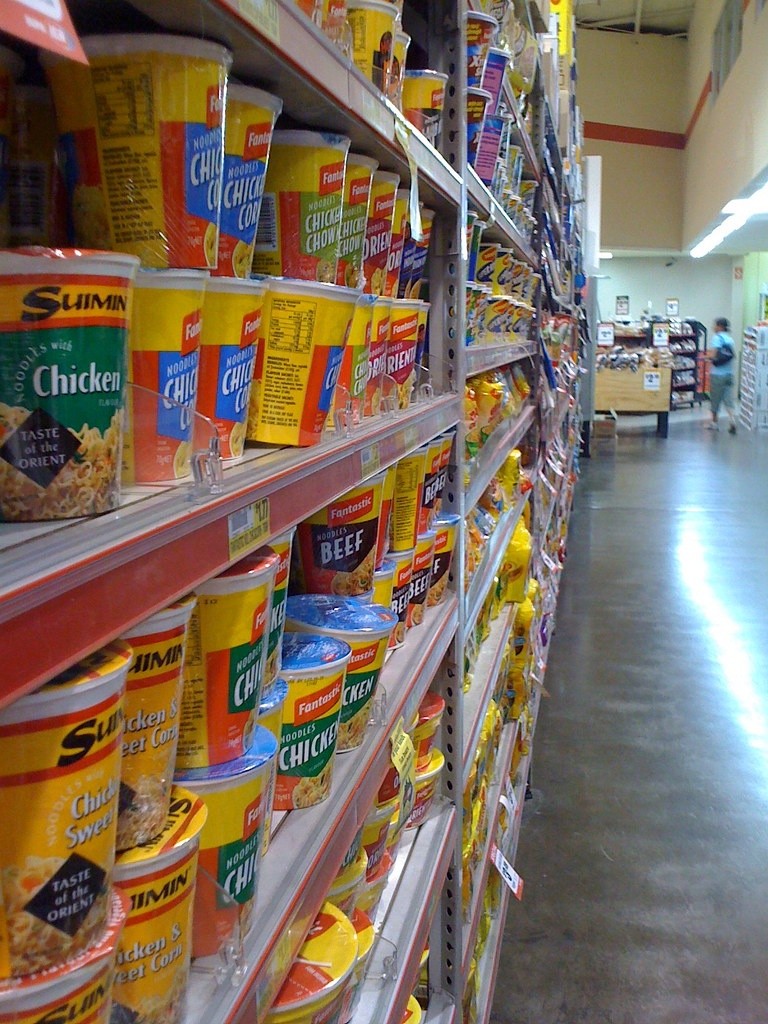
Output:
[594,407,618,439]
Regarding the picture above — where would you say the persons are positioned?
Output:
[704,318,736,433]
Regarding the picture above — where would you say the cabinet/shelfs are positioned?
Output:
[594,316,708,439]
[738,319,768,433]
[1,0,592,1024]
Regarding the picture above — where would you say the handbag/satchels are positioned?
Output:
[712,333,734,367]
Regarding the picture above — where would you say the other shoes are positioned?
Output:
[728,422,736,434]
[705,422,720,431]
